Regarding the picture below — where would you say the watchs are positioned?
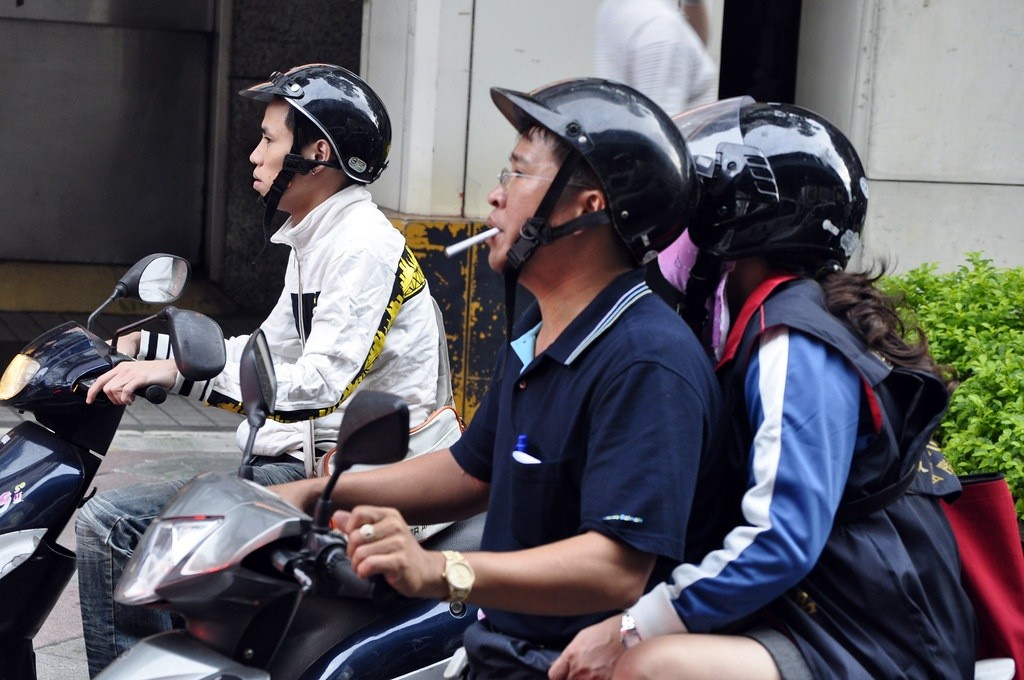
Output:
[439,549,476,605]
[618,608,644,649]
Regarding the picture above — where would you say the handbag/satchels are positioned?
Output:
[935,476,1023,680]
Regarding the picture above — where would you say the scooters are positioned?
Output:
[87,328,414,680]
[0,251,230,680]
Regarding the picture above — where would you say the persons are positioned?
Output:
[545,107,983,680]
[254,78,742,679]
[591,0,728,367]
[73,59,456,680]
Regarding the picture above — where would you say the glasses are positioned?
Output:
[497,168,595,191]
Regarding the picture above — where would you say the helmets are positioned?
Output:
[238,64,392,186]
[491,78,699,264]
[670,94,870,281]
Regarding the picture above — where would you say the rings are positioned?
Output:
[360,522,378,542]
